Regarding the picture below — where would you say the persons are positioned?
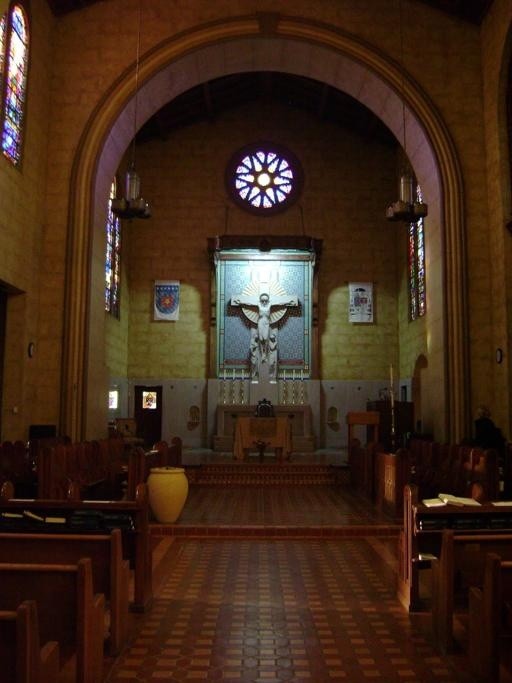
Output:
[233,292,296,360]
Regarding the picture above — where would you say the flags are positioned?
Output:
[153,278,180,322]
[348,281,374,323]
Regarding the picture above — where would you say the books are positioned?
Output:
[457,497,481,506]
[23,506,45,520]
[418,553,438,561]
[437,493,463,506]
[422,498,445,508]
[1,512,24,518]
[45,514,67,523]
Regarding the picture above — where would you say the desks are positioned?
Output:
[233,416,291,460]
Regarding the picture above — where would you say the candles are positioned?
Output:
[223,368,244,381]
[283,369,304,381]
[390,364,393,389]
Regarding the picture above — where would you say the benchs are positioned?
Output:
[0,434,184,503]
[1,483,153,615]
[367,438,512,683]
[2,556,106,681]
[3,598,62,681]
[2,526,133,654]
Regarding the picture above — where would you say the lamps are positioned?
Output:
[110,1,152,218]
[383,0,428,223]
